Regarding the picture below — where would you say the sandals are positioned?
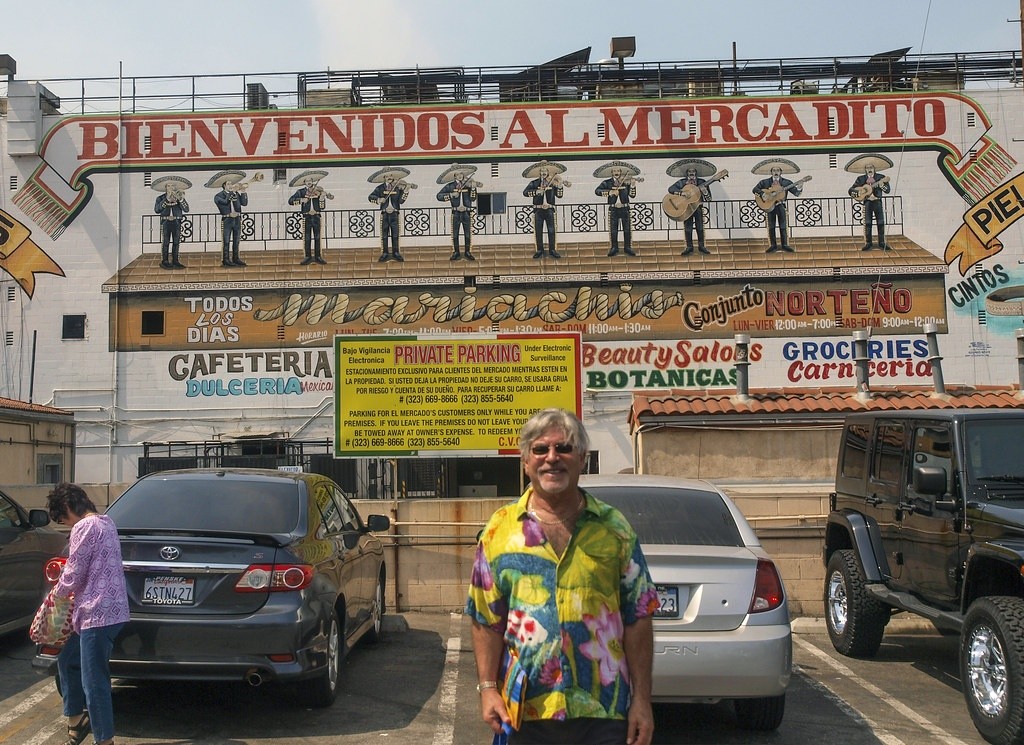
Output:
[60,713,91,745]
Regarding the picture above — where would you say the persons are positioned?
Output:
[466,409,660,745]
[48,483,131,745]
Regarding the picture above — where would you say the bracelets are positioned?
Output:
[477,681,498,695]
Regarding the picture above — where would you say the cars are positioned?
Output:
[0,489,70,638]
[31,466,391,710]
[475,472,793,733]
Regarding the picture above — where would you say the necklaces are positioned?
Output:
[530,492,585,525]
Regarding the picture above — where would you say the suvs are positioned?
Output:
[818,408,1024,745]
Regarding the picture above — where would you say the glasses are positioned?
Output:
[529,443,581,456]
